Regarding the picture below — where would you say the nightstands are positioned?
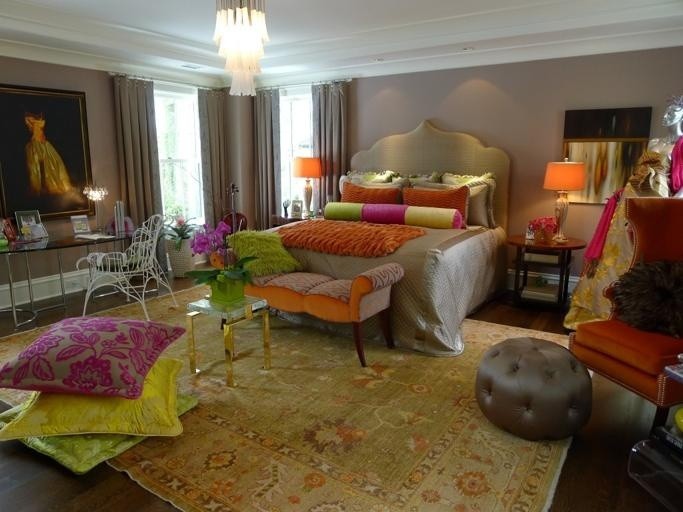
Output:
[270,210,314,227]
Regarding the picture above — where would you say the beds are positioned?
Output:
[261,117,511,342]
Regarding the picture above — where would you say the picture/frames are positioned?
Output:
[563,138,647,206]
[1,82,96,224]
[289,200,301,219]
[14,210,44,241]
[70,215,90,233]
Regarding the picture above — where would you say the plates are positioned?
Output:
[554,239,569,244]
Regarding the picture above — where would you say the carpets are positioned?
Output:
[0,280,601,510]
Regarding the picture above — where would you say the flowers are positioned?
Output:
[527,214,557,234]
[162,212,197,251]
[184,224,260,283]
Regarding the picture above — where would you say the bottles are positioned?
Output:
[0,223,8,249]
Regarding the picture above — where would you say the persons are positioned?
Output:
[25,114,71,195]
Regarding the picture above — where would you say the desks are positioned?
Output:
[1,228,141,329]
[621,356,681,512]
[504,233,588,314]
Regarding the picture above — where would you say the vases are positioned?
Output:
[208,278,246,305]
[534,231,554,242]
[165,237,192,278]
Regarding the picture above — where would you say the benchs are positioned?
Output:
[210,250,404,368]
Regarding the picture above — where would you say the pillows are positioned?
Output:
[1,395,198,476]
[0,353,183,449]
[323,166,496,231]
[228,230,302,280]
[1,315,186,399]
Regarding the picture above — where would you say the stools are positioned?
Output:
[184,294,274,387]
[476,337,592,446]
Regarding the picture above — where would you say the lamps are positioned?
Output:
[293,155,324,214]
[541,154,586,245]
[84,183,109,231]
[211,0,271,97]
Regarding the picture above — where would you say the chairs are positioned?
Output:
[75,211,178,322]
[561,197,683,481]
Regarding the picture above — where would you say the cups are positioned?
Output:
[304,210,309,218]
[309,211,314,217]
